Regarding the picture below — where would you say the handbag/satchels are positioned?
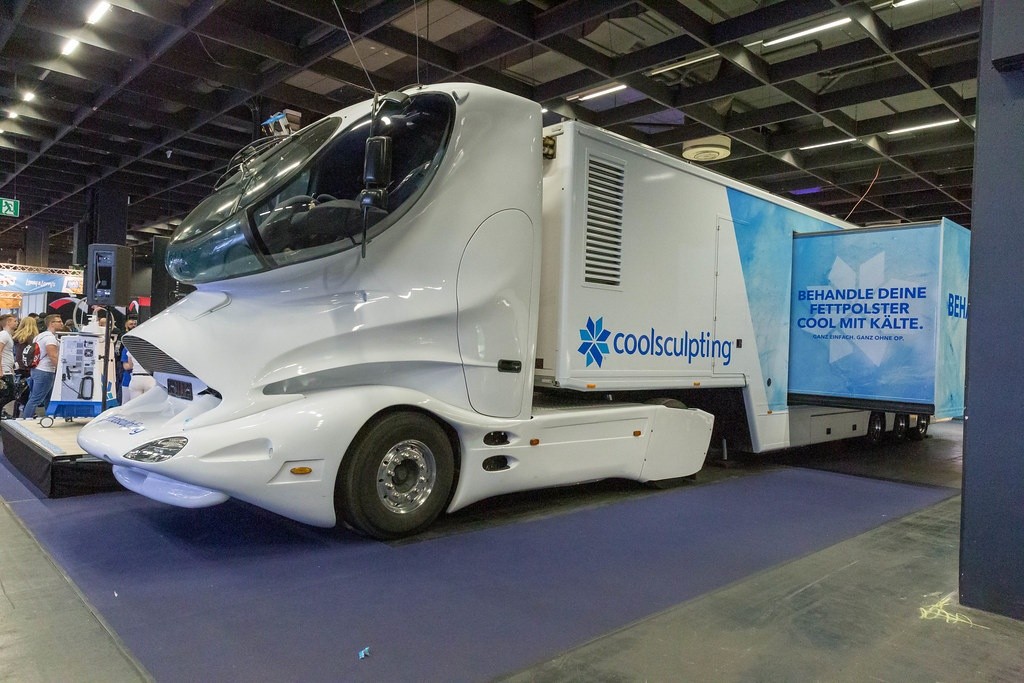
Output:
[13,378,31,404]
[0,376,19,399]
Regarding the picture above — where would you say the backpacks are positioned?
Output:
[22,334,56,368]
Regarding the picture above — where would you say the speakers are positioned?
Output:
[87,242,132,307]
[149,234,188,305]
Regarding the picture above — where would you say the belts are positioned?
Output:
[132,373,152,376]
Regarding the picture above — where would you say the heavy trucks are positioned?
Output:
[73,78,968,543]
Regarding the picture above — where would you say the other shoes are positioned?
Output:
[1,411,12,419]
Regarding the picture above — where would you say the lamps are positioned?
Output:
[681,134,732,162]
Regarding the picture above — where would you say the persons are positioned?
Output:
[99,313,158,406]
[0,313,78,420]
